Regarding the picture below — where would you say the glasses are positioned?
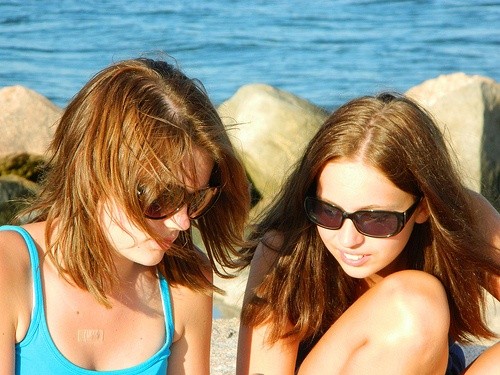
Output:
[134,181,224,221]
[303,177,423,239]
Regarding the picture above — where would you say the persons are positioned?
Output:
[232,90,500,375]
[0,58,251,374]
